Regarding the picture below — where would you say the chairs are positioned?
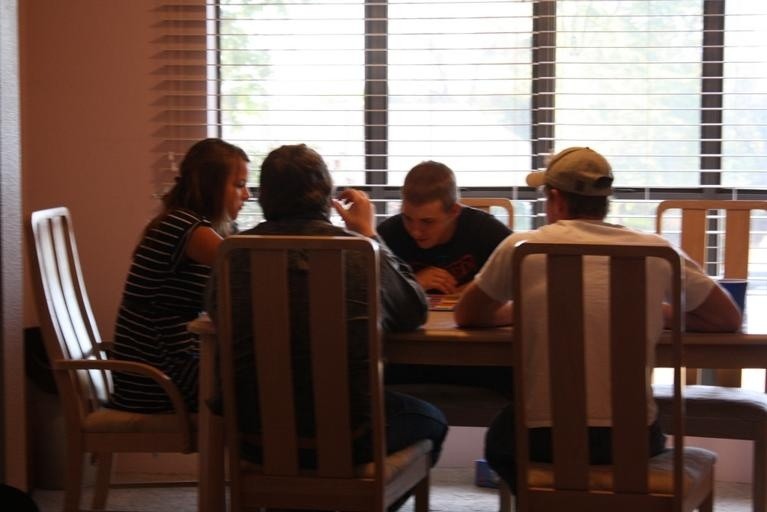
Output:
[511,240,719,512]
[461,198,513,231]
[27,207,200,511]
[216,234,434,511]
[654,198,767,511]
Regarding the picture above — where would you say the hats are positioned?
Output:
[527,146,614,199]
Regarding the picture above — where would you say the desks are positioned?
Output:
[184,281,766,511]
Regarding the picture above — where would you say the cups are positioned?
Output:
[715,279,748,313]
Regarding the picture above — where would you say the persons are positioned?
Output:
[204,144,447,469]
[453,149,743,500]
[377,159,517,292]
[114,137,252,411]
